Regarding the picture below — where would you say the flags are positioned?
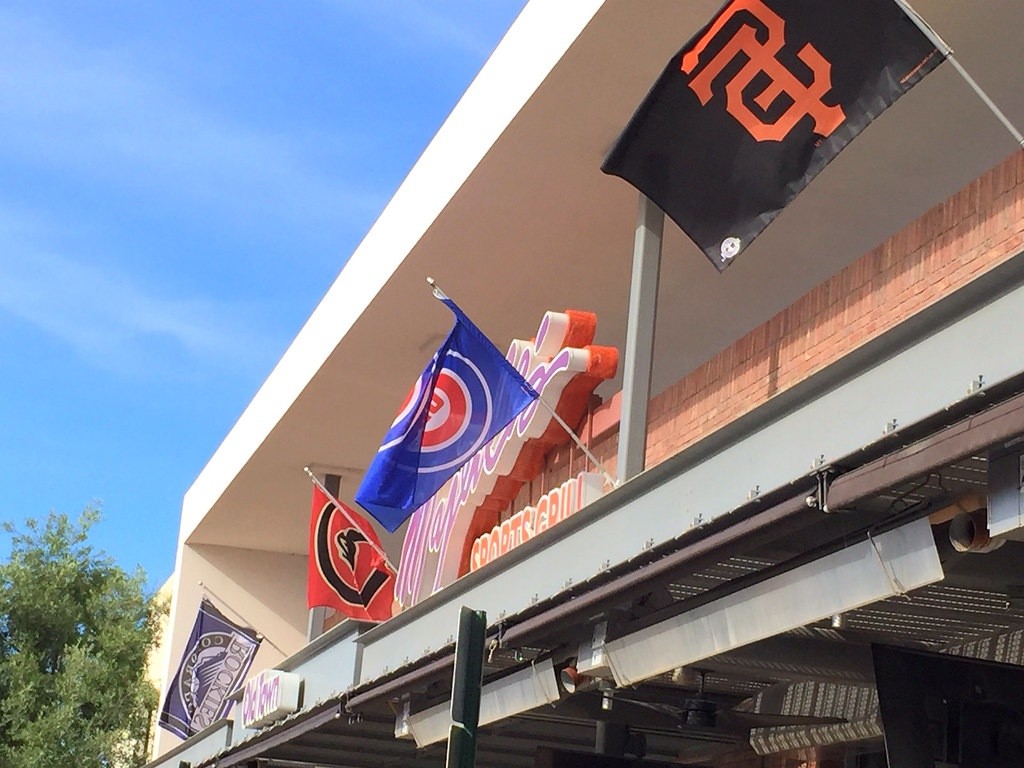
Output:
[159,597,264,741]
[601,1,954,275]
[354,289,541,533]
[306,477,398,623]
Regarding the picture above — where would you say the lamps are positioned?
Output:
[559,666,584,695]
[686,667,717,728]
